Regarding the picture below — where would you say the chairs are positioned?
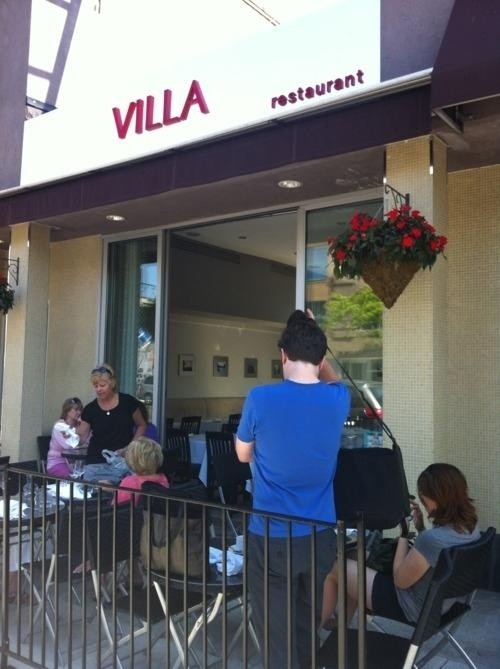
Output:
[308,526,498,669]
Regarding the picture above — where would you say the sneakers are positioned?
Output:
[317,613,338,639]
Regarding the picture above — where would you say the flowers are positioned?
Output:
[325,205,449,284]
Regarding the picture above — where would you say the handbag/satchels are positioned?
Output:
[334,442,411,531]
[365,538,397,574]
[140,510,203,578]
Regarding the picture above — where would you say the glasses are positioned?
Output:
[71,397,79,403]
[90,366,112,376]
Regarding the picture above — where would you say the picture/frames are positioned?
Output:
[178,353,285,379]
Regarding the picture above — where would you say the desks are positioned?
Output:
[139,533,369,669]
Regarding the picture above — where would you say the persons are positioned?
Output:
[60,364,149,487]
[316,463,483,639]
[70,436,172,604]
[132,401,160,443]
[231,307,354,668]
[44,397,89,480]
